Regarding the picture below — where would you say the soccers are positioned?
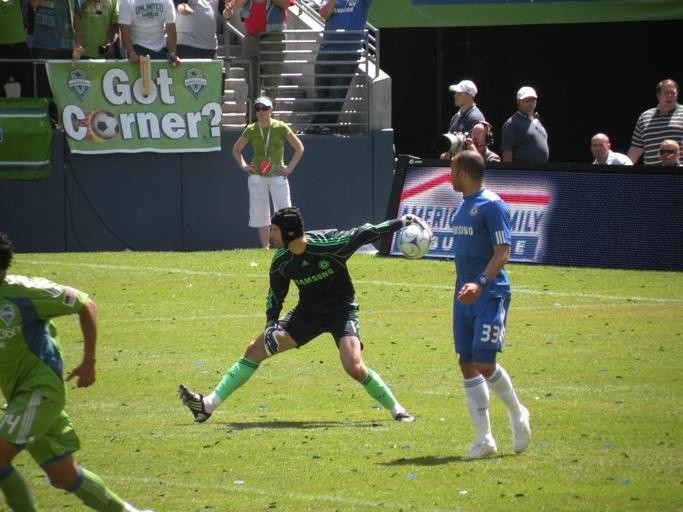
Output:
[91,111,120,139]
[397,219,433,259]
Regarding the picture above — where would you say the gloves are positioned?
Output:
[401,213,430,236]
[263,321,287,356]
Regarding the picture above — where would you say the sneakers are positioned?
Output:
[510,404,531,453]
[395,409,415,422]
[463,439,497,459]
[178,384,210,423]
[304,127,321,133]
[320,126,332,133]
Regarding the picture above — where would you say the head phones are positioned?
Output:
[477,121,495,144]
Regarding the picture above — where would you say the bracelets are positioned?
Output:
[474,273,488,290]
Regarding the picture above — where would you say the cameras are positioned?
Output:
[99,44,109,54]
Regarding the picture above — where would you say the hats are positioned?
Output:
[516,86,537,100]
[449,79,478,97]
[252,96,272,106]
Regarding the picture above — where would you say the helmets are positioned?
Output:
[271,207,304,244]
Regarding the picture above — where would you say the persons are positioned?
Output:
[234,1,296,123]
[658,139,683,167]
[232,96,305,249]
[0,1,234,130]
[309,1,370,134]
[439,121,501,163]
[447,80,485,134]
[179,206,433,424]
[500,86,550,163]
[591,133,633,166]
[626,79,683,167]
[0,231,154,512]
[449,149,532,461]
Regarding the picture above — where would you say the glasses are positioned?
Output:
[660,149,673,154]
[255,106,270,110]
